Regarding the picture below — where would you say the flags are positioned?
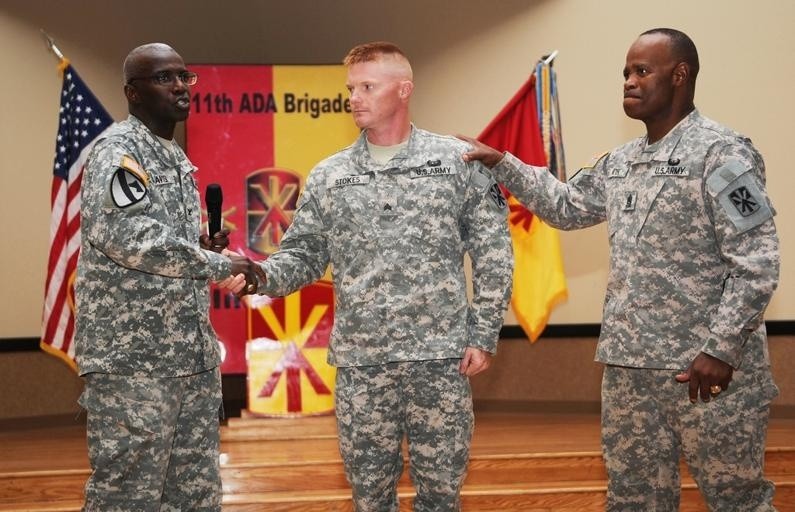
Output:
[40,59,114,374]
[477,63,568,345]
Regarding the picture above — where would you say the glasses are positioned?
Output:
[127,69,199,90]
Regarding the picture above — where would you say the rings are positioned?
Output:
[245,283,254,292]
[710,385,721,393]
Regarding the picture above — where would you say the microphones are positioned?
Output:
[205,184,223,247]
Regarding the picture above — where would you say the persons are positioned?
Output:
[456,29,781,512]
[214,42,516,512]
[74,42,267,512]
